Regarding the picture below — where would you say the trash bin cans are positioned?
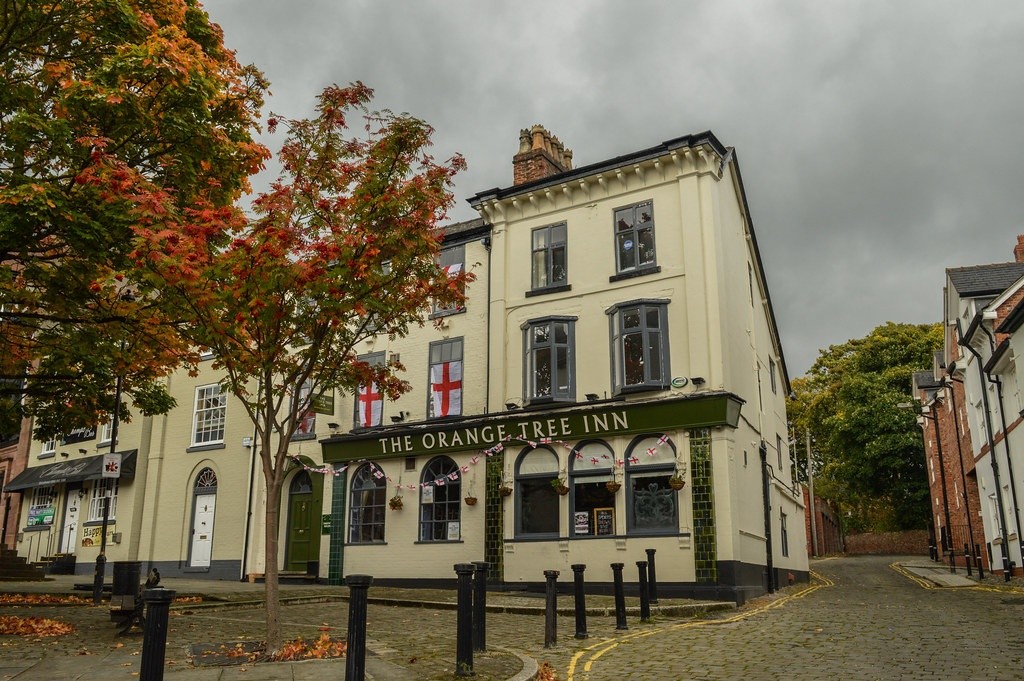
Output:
[112,561,143,595]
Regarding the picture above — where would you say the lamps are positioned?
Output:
[79,449,87,454]
[585,393,600,401]
[60,452,69,458]
[691,377,705,386]
[505,403,519,410]
[78,486,88,501]
[48,486,57,503]
[390,416,402,423]
[327,422,340,429]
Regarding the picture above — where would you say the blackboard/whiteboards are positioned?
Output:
[594,508,615,535]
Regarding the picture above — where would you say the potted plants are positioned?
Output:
[498,480,515,496]
[669,460,687,490]
[606,473,624,492]
[551,479,570,496]
[464,492,477,505]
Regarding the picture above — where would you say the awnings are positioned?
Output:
[3,449,138,494]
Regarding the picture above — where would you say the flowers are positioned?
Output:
[388,496,403,512]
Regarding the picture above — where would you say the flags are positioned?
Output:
[297,389,314,433]
[515,435,669,466]
[370,434,511,491]
[438,263,461,289]
[434,361,461,417]
[359,380,382,427]
[304,459,366,477]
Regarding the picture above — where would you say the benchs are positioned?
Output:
[109,567,161,636]
